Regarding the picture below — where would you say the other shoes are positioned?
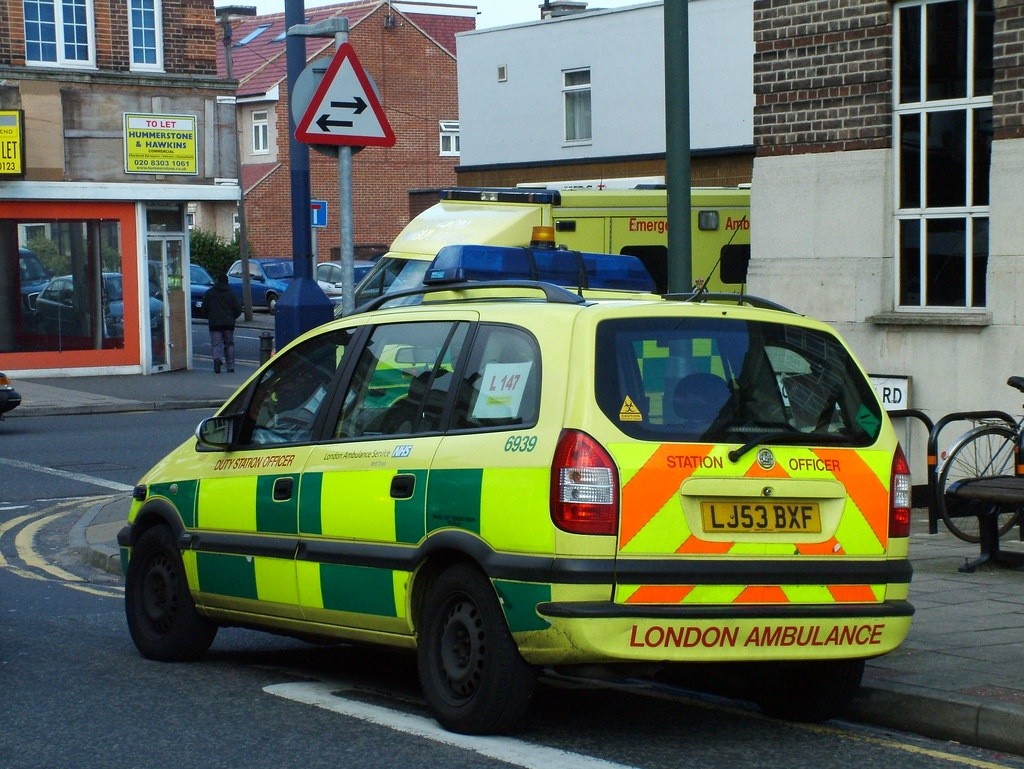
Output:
[228,369,235,372]
[214,359,223,374]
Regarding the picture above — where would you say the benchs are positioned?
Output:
[946,476,1024,574]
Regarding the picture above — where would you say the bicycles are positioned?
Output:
[935,376,1024,544]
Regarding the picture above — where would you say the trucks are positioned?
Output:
[335,182,752,317]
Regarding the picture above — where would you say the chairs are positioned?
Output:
[378,369,450,436]
[673,373,733,423]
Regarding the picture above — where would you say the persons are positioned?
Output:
[149,264,164,303]
[200,274,242,372]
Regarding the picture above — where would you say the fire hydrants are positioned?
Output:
[258,332,275,366]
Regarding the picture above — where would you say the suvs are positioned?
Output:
[18,249,50,328]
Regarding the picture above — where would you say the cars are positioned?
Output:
[35,272,164,343]
[0,372,21,415]
[227,259,294,314]
[116,227,916,736]
[314,260,403,306]
[190,264,215,319]
